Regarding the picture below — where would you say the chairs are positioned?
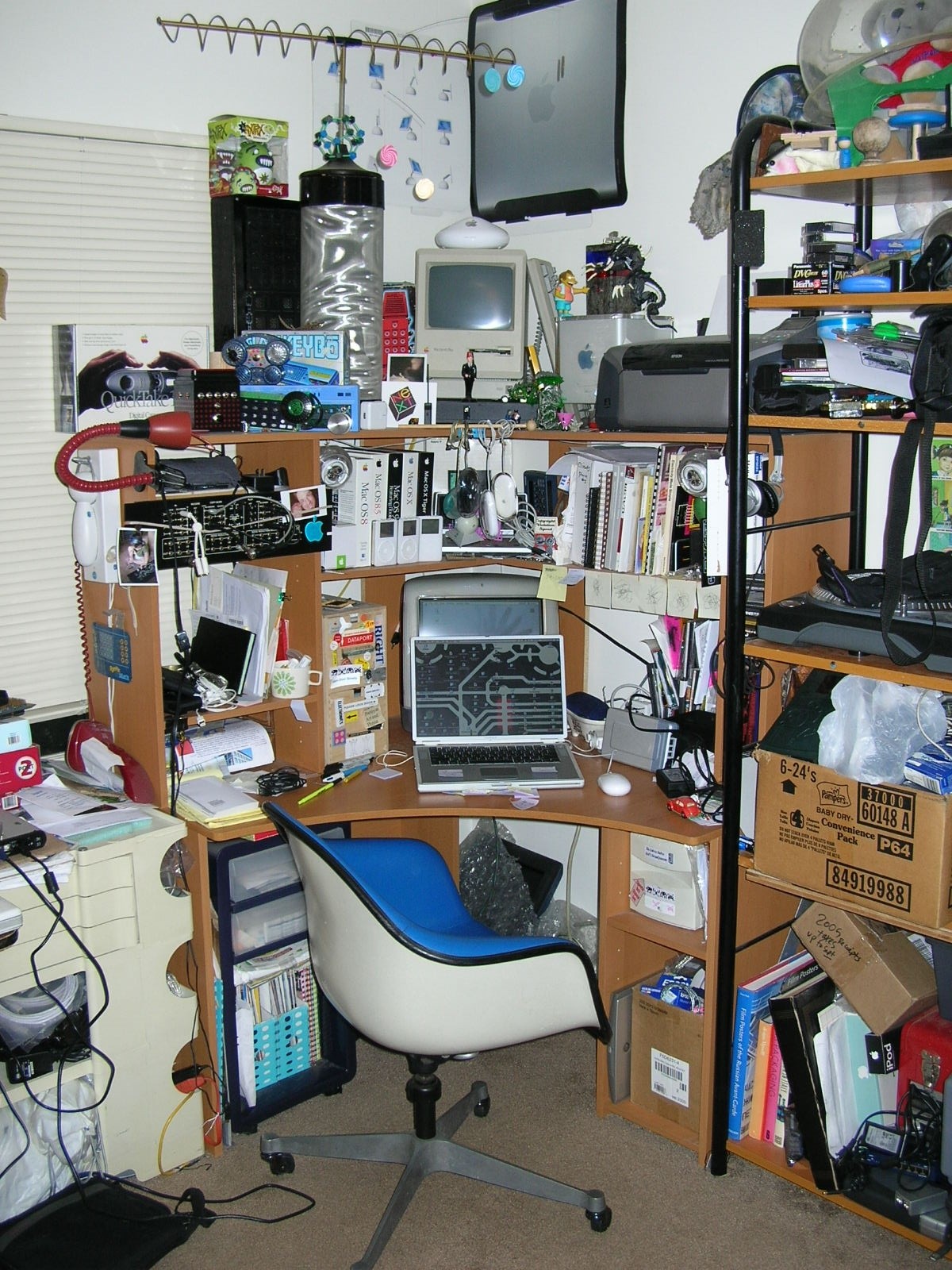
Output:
[261,802,618,1270]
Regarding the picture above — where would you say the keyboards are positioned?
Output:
[526,259,560,373]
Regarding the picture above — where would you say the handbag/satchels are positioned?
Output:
[910,304,952,419]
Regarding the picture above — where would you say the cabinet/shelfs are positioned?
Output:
[0,108,952,1270]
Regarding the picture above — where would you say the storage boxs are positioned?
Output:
[207,113,288,198]
[793,263,852,294]
[631,974,706,1129]
[237,329,349,384]
[754,746,952,928]
[792,902,937,1035]
[865,1032,900,1074]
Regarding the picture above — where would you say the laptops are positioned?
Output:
[409,635,586,793]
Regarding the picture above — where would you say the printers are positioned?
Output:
[595,315,825,434]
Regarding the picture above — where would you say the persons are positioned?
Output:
[291,489,316,518]
[78,349,203,416]
[461,351,477,400]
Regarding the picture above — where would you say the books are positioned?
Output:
[233,938,321,1107]
[719,953,899,1166]
[170,774,259,819]
[183,564,288,708]
[319,438,766,576]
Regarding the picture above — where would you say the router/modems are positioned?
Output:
[601,708,680,773]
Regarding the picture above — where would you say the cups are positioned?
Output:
[271,660,323,699]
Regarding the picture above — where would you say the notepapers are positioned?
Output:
[369,768,403,779]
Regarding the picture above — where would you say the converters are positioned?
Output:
[655,767,696,798]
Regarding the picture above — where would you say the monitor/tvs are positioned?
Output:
[400,572,560,733]
[415,249,527,380]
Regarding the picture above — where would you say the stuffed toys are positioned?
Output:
[759,140,799,176]
[860,0,952,108]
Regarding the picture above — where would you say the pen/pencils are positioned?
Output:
[323,765,368,782]
[298,778,342,804]
[344,770,362,782]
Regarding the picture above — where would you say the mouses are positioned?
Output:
[597,772,632,797]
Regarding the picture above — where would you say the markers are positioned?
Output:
[323,754,373,772]
[320,759,372,779]
